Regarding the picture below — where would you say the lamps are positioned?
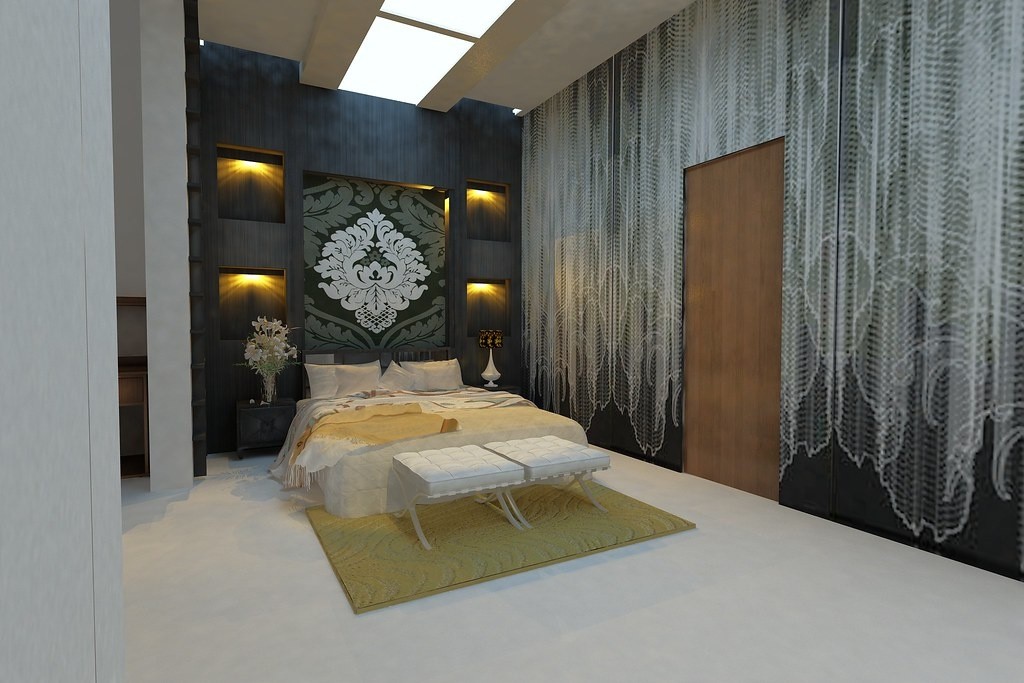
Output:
[477,329,506,388]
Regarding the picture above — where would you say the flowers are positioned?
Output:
[241,315,300,374]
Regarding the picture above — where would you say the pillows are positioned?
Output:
[304,359,383,400]
[399,357,464,391]
[379,360,417,392]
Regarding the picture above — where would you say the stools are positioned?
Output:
[390,435,612,551]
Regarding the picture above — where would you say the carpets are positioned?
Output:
[303,473,696,615]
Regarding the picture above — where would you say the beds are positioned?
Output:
[273,346,589,519]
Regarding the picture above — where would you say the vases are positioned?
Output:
[260,372,281,407]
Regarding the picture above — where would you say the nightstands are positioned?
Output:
[468,384,522,400]
[236,398,297,461]
